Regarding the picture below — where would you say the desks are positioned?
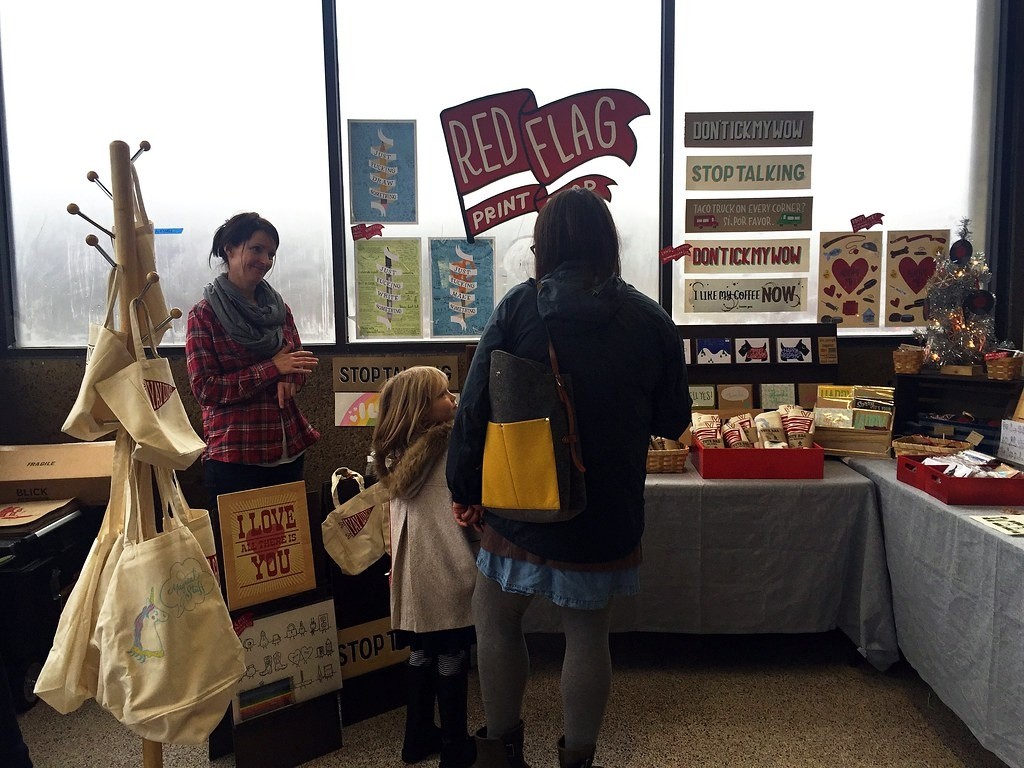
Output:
[840,453,1024,766]
[893,372,1024,456]
[465,455,899,675]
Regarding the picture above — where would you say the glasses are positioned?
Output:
[531,244,536,254]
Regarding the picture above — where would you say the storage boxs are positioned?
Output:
[813,406,896,459]
[0,523,95,642]
[690,434,825,479]
[0,441,115,507]
[896,452,1024,505]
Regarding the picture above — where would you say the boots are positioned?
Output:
[556,735,602,768]
[472,719,529,768]
[437,658,487,768]
[402,658,441,764]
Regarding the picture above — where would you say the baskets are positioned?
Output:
[986,357,1022,381]
[646,444,689,473]
[892,436,971,458]
[892,351,922,375]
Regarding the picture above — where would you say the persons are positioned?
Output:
[186,212,322,495]
[370,365,484,768]
[445,187,693,768]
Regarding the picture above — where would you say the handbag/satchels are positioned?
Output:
[479,325,586,522]
[93,297,207,471]
[32,426,246,744]
[59,262,135,441]
[115,158,172,346]
[322,467,391,576]
